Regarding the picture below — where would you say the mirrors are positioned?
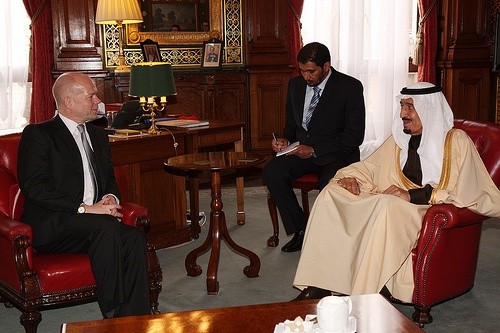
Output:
[124,0,224,47]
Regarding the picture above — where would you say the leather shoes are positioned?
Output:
[282,230,305,252]
[288,288,333,302]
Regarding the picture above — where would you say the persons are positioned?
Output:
[201,19,209,32]
[16,73,151,318]
[172,25,179,31]
[265,43,366,253]
[292,81,500,300]
[149,47,158,61]
[208,46,217,62]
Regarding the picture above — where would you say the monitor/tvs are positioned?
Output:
[109,99,142,129]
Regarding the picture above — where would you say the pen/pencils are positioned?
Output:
[272,133,281,151]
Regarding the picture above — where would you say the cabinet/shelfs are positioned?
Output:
[36,60,295,185]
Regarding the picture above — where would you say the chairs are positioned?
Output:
[371,121,500,328]
[0,133,161,333]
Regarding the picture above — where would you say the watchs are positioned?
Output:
[78,205,86,213]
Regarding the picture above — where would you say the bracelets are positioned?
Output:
[312,149,316,157]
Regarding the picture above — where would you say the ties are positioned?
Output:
[306,87,321,129]
[77,125,98,205]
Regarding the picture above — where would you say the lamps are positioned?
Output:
[95,0,144,73]
[127,62,178,135]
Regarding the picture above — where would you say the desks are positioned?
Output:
[59,292,425,332]
[155,119,247,239]
[165,149,261,295]
[83,127,193,249]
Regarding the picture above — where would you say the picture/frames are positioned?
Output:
[147,1,199,32]
[139,38,162,64]
[200,38,224,69]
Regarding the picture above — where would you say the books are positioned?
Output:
[156,120,209,127]
[276,142,299,157]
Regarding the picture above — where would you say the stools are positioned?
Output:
[264,177,321,248]
